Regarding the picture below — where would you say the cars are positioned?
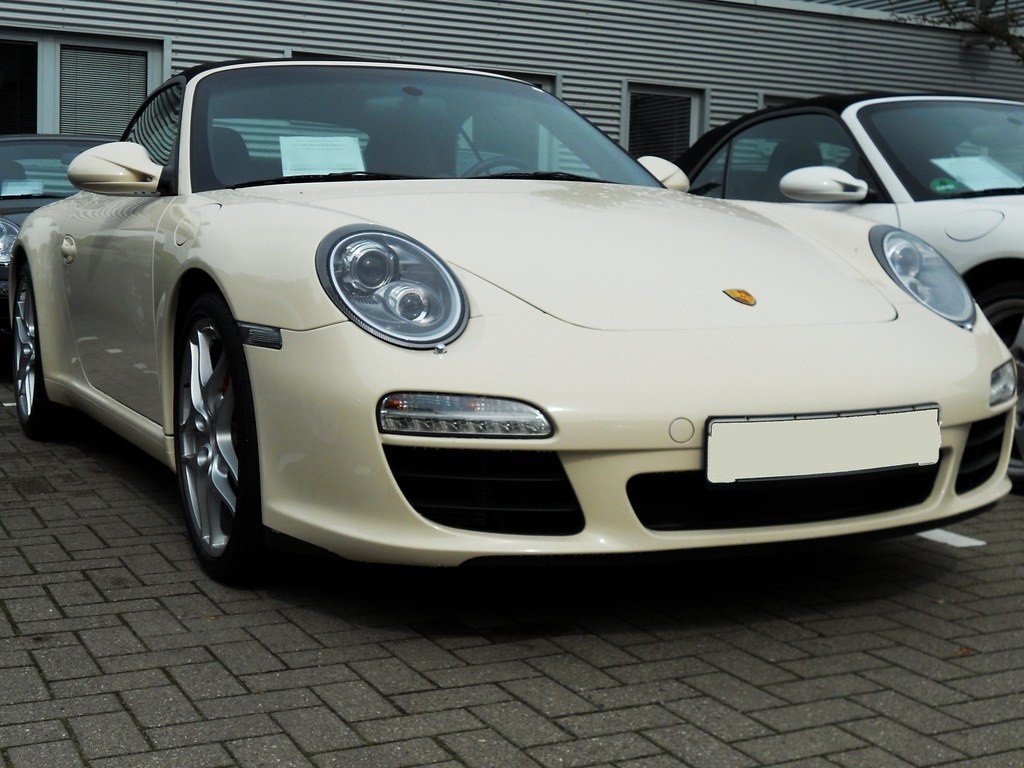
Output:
[0,132,123,348]
[677,90,1024,478]
[10,58,1020,594]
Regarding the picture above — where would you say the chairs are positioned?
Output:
[166,126,272,187]
[757,140,824,204]
